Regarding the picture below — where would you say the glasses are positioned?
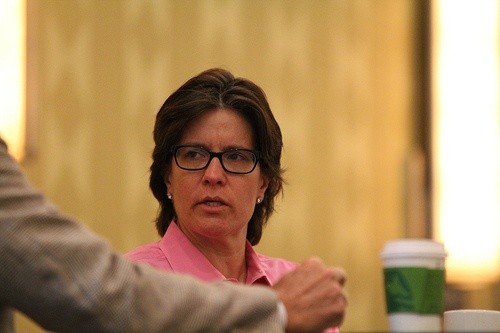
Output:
[172,145,260,174]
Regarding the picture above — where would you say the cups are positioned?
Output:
[379,239,448,333]
[442,309,500,333]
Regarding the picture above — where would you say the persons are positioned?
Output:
[0,134,348,333]
[123,66,342,333]
